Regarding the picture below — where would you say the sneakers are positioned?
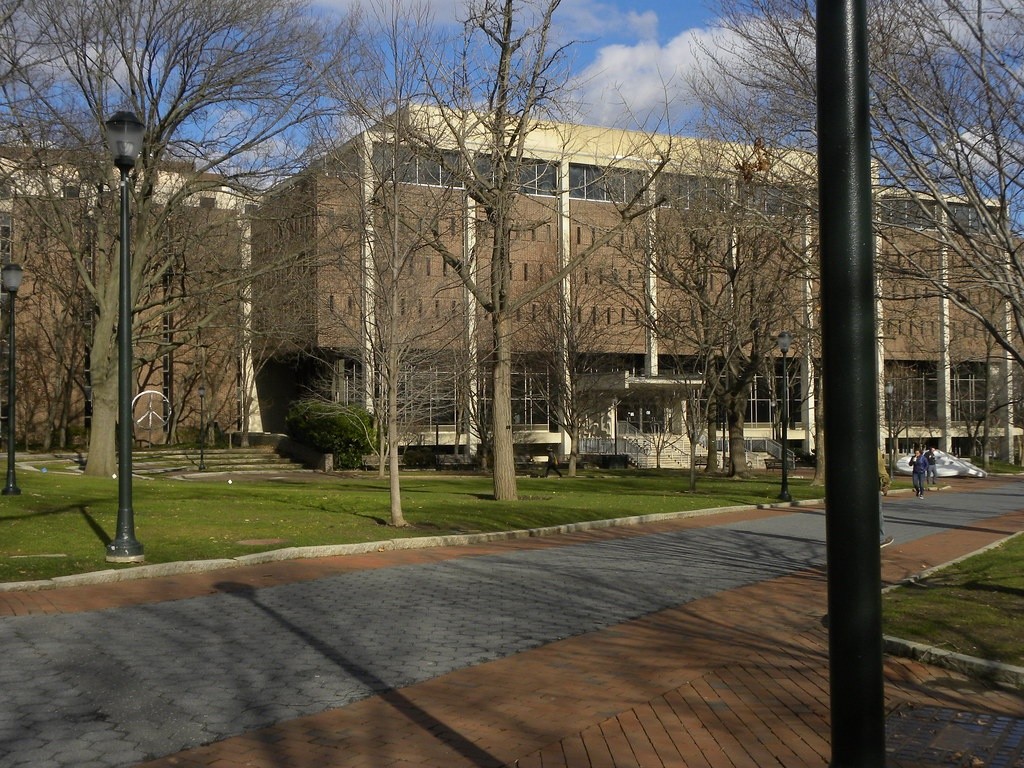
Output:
[880,536,894,548]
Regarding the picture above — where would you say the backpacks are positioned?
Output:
[554,454,558,466]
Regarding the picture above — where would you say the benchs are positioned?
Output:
[765,459,793,474]
[724,456,730,467]
[695,456,708,468]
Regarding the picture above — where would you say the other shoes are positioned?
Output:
[916,491,924,499]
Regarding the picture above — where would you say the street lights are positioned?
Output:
[102,109,150,565]
[884,380,895,481]
[1,262,24,497]
[197,385,205,472]
[776,331,794,502]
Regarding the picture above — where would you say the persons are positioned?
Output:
[878,447,894,549]
[544,446,563,479]
[923,447,937,486]
[908,449,929,499]
